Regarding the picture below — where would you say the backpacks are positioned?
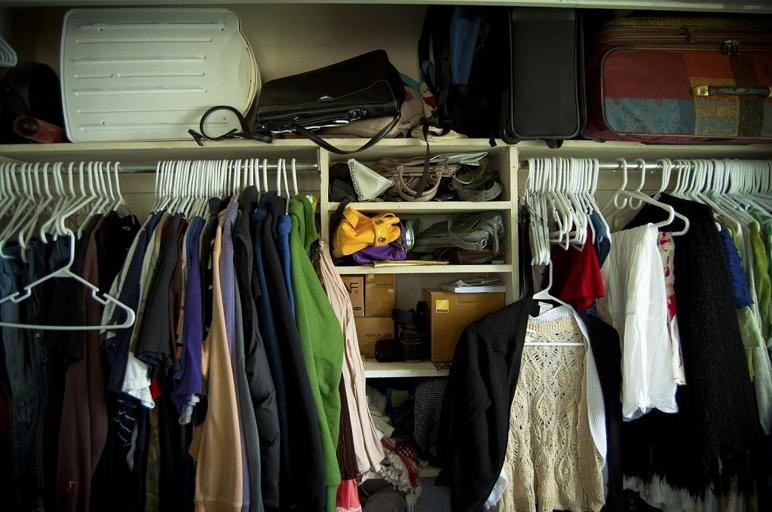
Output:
[419,4,508,135]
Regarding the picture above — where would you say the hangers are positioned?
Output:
[522,256,587,347]
[0,159,300,264]
[0,229,136,330]
[519,157,772,266]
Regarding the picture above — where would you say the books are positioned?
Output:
[440,276,506,293]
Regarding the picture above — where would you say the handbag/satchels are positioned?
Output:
[255,49,405,154]
[333,206,506,263]
[347,152,504,201]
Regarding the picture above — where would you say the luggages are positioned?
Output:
[580,16,771,146]
[500,7,582,149]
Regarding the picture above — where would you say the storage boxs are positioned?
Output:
[422,286,506,363]
[58,6,261,141]
